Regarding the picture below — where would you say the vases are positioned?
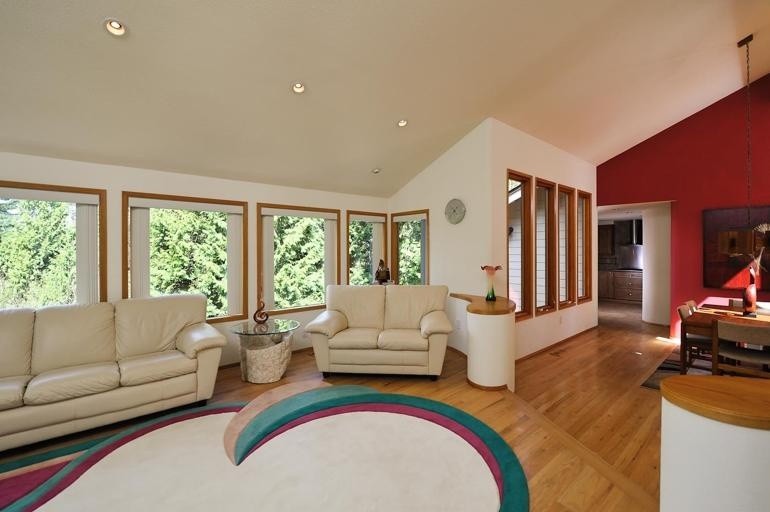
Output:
[743,266,758,317]
[481,265,503,301]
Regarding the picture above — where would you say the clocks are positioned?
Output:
[444,200,466,224]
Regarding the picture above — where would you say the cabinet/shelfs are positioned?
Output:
[614,272,642,304]
[598,271,615,302]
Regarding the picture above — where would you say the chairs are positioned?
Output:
[676,299,770,379]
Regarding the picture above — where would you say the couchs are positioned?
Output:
[305,285,453,381]
[1,293,228,454]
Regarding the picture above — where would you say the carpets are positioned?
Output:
[0,381,530,512]
[639,345,740,393]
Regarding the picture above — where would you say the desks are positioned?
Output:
[658,374,770,511]
